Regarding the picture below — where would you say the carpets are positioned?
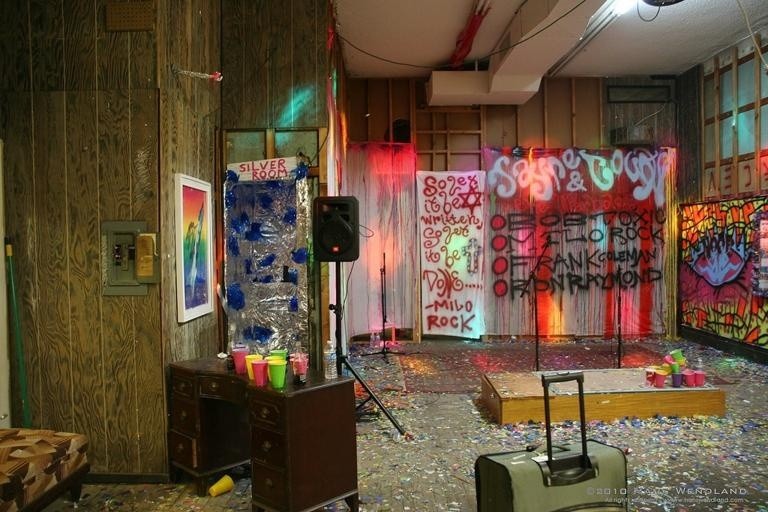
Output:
[346,342,672,396]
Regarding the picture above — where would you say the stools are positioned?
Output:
[1,428,89,512]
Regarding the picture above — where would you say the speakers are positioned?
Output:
[314,196,359,262]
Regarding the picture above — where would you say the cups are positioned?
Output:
[645,349,706,388]
[231,346,288,389]
[290,352,308,373]
[209,474,235,498]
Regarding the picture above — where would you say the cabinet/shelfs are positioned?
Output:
[166,355,359,512]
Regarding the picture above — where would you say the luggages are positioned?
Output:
[475,370,627,512]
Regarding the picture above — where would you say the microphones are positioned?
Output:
[546,235,552,242]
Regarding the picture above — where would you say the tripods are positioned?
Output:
[358,265,410,364]
[312,270,411,441]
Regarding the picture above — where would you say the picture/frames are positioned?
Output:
[175,173,215,325]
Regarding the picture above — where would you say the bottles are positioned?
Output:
[292,341,308,385]
[322,340,338,379]
